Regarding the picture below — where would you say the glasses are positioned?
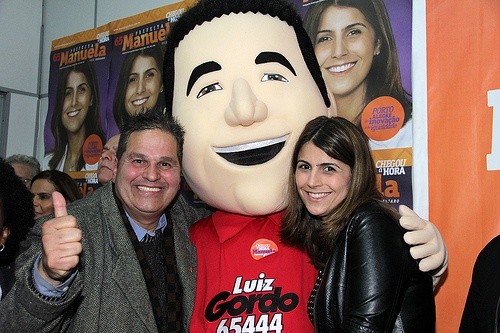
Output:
[22,178,32,184]
